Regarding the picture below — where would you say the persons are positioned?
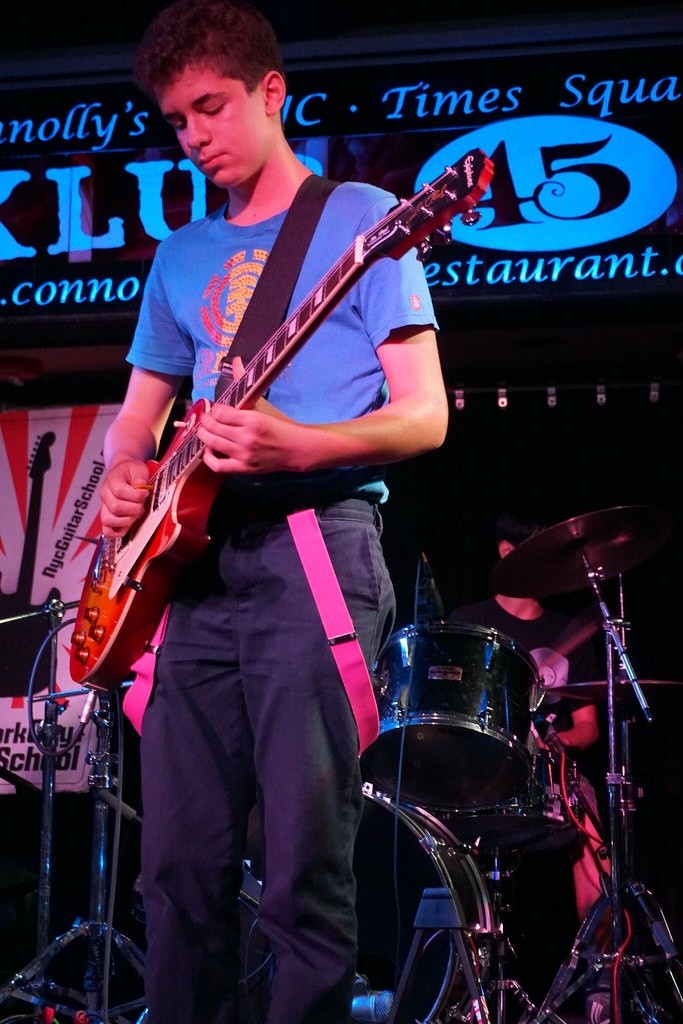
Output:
[407,498,642,1024]
[100,0,449,1024]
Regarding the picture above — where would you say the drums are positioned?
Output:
[441,745,586,862]
[358,619,547,815]
[237,781,497,1024]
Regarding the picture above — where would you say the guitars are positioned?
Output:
[67,146,497,697]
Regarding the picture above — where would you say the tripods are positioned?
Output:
[0,600,152,1024]
[442,542,683,1024]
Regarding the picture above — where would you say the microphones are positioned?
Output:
[534,715,566,756]
[418,551,446,621]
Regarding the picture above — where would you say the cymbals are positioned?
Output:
[541,678,683,703]
[493,504,678,599]
